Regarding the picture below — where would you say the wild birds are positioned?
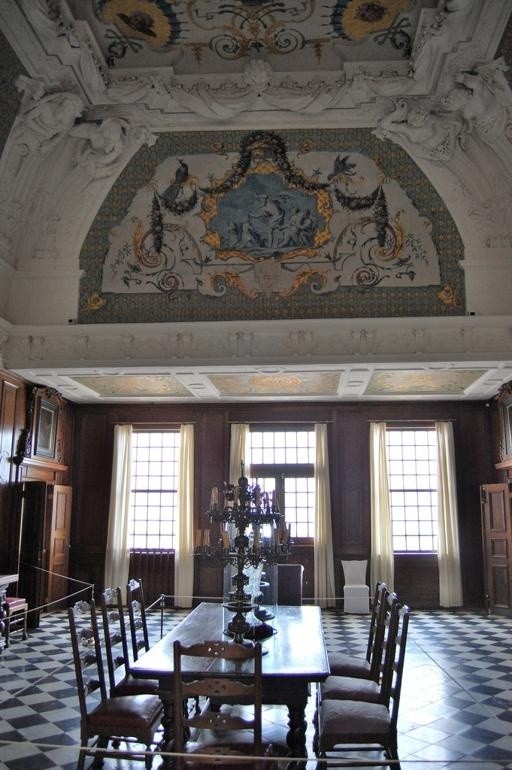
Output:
[160,157,189,200]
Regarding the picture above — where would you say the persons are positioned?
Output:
[82,114,126,169]
[376,67,509,152]
[15,95,85,139]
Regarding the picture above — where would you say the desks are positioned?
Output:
[129,601,324,770]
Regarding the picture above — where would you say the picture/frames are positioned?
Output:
[34,396,59,459]
[502,396,512,455]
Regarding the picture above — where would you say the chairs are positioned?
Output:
[125,577,202,715]
[312,592,400,729]
[254,565,306,605]
[101,587,191,749]
[317,581,390,709]
[71,596,162,770]
[5,597,28,648]
[312,606,408,770]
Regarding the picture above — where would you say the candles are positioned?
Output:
[195,487,291,548]
